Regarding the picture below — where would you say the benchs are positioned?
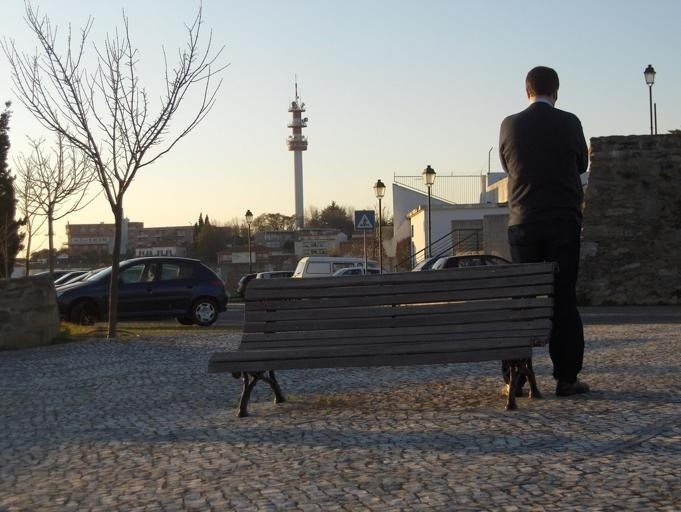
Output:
[207,259,559,418]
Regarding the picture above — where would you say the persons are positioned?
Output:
[498,66,590,396]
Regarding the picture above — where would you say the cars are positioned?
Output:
[237,252,517,298]
[29,254,228,328]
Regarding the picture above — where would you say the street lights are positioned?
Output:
[243,209,258,273]
[371,178,393,273]
[641,62,662,134]
[421,164,436,257]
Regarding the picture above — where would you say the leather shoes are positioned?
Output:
[556,380,589,396]
[502,383,522,397]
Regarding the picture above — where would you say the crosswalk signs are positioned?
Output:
[354,209,375,230]
